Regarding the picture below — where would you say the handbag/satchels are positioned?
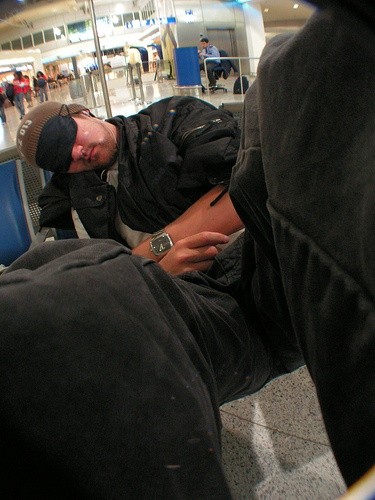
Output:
[233,74,249,94]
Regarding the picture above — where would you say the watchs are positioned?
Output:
[149,229,173,257]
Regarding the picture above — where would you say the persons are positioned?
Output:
[12,71,25,118]
[198,37,220,86]
[35,71,49,101]
[154,54,161,80]
[1,236,230,500]
[0,86,7,125]
[48,72,68,87]
[173,0,375,488]
[14,102,243,279]
[24,75,32,106]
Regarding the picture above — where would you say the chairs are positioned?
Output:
[202,50,239,94]
[0,101,244,268]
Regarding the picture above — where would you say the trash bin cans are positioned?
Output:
[173,46,202,85]
[69,78,86,99]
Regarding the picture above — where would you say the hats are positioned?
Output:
[16,102,96,166]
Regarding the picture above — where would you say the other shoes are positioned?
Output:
[27,102,33,107]
[209,83,216,87]
[2,122,6,125]
[40,101,42,103]
[45,101,48,102]
[19,114,24,118]
